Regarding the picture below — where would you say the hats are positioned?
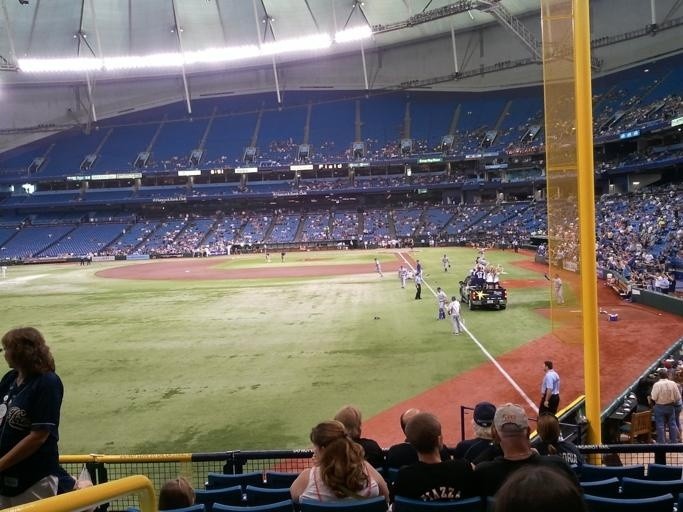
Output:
[494,403,528,432]
[474,402,496,427]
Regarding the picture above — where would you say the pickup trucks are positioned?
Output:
[458,275,508,311]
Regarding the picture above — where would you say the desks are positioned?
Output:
[640,349,683,406]
[607,392,638,443]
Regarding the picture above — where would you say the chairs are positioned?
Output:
[126,450,683,511]
[620,410,653,444]
[538,175,683,294]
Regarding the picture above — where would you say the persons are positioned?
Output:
[447,296,463,335]
[158,479,194,511]
[399,260,422,299]
[289,400,589,512]
[443,254,450,272]
[374,257,383,277]
[1,88,683,269]
[650,342,683,444]
[539,361,560,415]
[436,287,447,321]
[0,327,64,507]
[552,273,565,304]
[631,270,676,294]
[476,257,500,283]
